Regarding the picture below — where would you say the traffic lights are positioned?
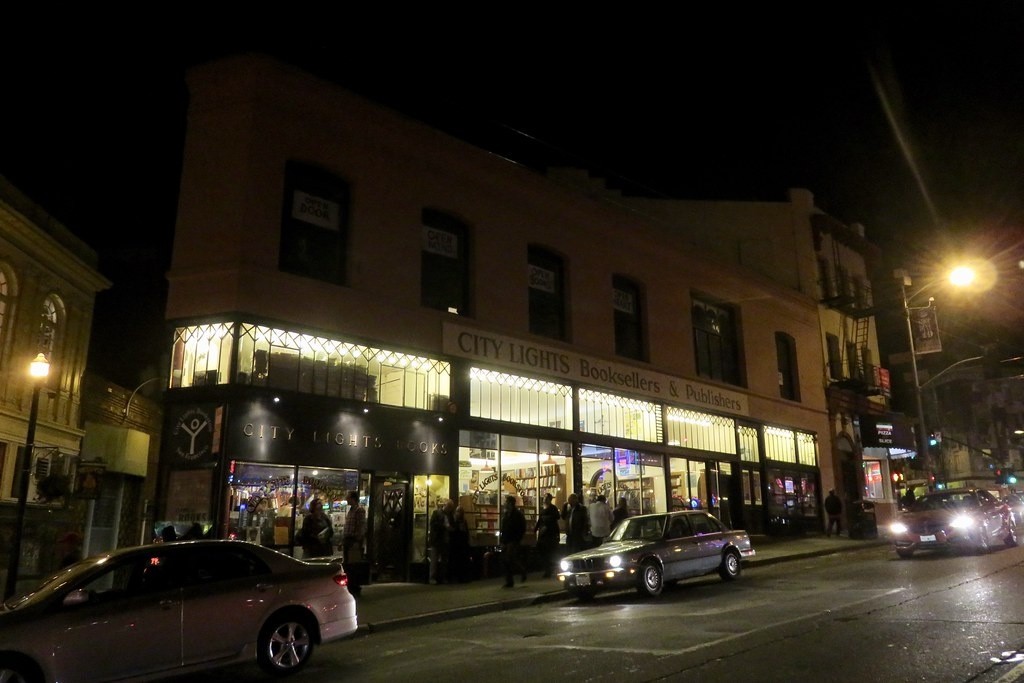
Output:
[1005,468,1017,485]
[995,470,1004,485]
[928,432,937,447]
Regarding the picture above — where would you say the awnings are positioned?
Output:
[858,412,918,460]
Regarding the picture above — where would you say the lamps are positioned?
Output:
[481,461,493,471]
[543,455,557,465]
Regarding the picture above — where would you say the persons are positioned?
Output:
[610,498,628,532]
[501,496,526,588]
[429,498,470,585]
[534,492,560,579]
[825,488,842,537]
[906,485,915,504]
[300,498,334,558]
[589,495,614,546]
[561,493,589,555]
[337,490,367,597]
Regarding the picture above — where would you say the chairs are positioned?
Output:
[695,522,710,534]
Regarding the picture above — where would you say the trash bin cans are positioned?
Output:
[847,501,878,540]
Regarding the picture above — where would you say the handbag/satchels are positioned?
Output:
[336,560,372,586]
[295,526,319,548]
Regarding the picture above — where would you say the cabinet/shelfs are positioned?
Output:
[458,466,654,548]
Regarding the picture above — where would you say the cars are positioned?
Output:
[557,509,756,606]
[884,487,1021,560]
[0,538,358,683]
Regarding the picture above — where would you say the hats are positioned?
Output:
[569,494,578,501]
[829,488,835,495]
[56,532,84,546]
[542,493,557,500]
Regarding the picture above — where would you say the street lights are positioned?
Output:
[898,259,981,495]
[4,354,53,607]
